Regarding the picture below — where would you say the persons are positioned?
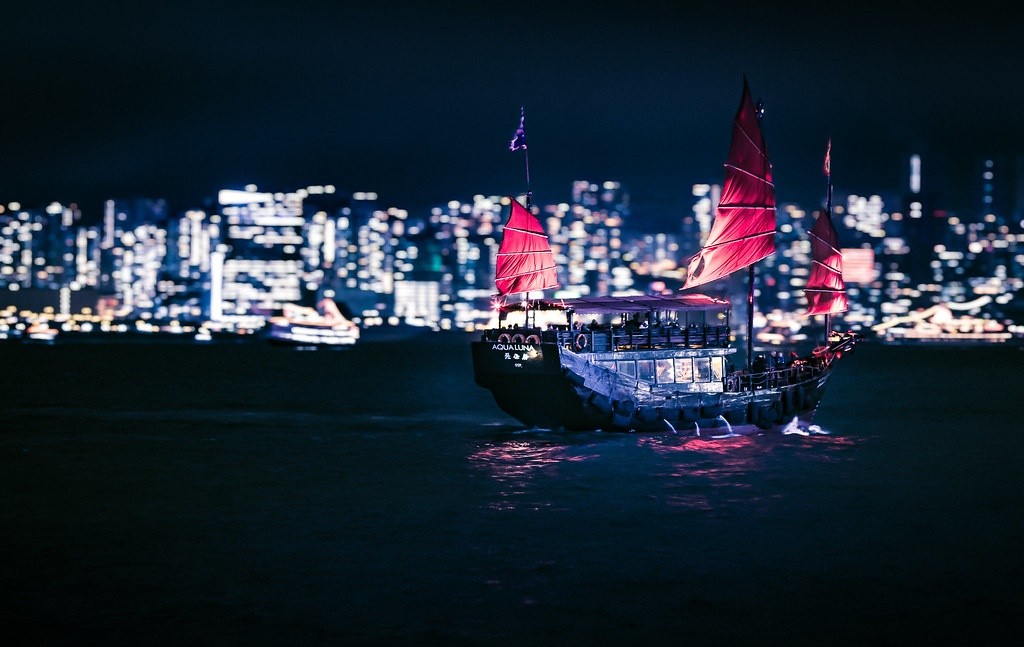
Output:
[573,319,601,331]
[547,324,569,332]
[623,313,656,329]
[751,351,800,390]
[502,324,518,329]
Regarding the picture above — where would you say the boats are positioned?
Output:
[869,294,1014,344]
[469,68,879,434]
[254,297,362,348]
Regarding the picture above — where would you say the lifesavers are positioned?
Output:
[525,335,540,345]
[576,334,587,349]
[511,334,525,344]
[498,333,511,344]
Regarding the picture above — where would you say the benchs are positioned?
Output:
[483,325,733,352]
